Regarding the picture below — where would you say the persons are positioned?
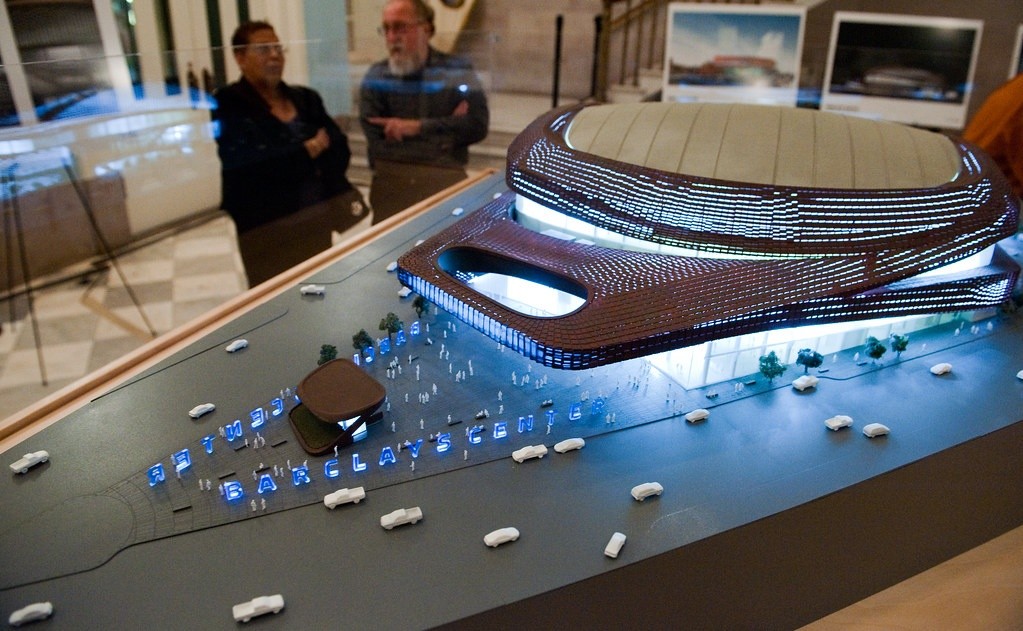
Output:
[960,72,1023,199]
[357,0,489,228]
[209,21,351,292]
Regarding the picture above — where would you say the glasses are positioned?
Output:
[246,42,291,58]
[375,17,427,39]
